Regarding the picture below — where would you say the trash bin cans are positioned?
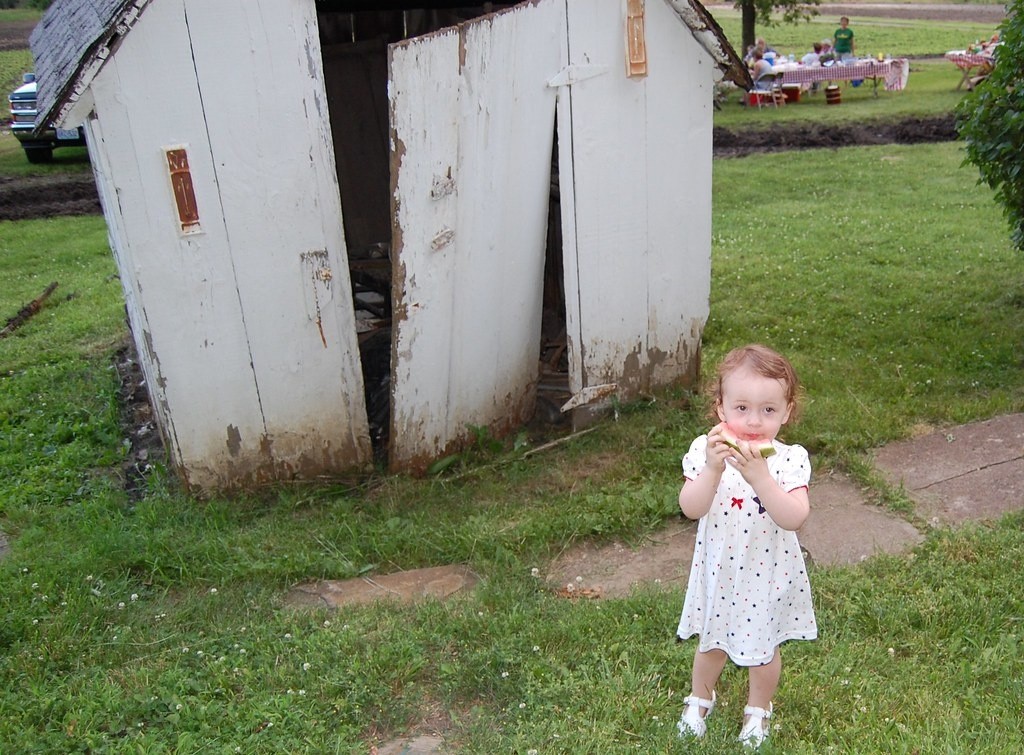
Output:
[825,85,840,104]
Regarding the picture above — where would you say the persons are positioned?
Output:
[830,16,856,61]
[676,343,817,752]
[812,38,832,88]
[749,49,773,91]
[742,38,781,66]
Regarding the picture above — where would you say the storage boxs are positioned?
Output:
[748,89,765,106]
[782,83,801,103]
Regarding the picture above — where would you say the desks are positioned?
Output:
[746,58,908,109]
[945,49,994,91]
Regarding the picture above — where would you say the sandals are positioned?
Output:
[676,689,716,742]
[739,699,773,751]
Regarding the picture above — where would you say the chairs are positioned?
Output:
[748,71,784,111]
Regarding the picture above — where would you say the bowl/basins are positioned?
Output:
[366,241,388,258]
